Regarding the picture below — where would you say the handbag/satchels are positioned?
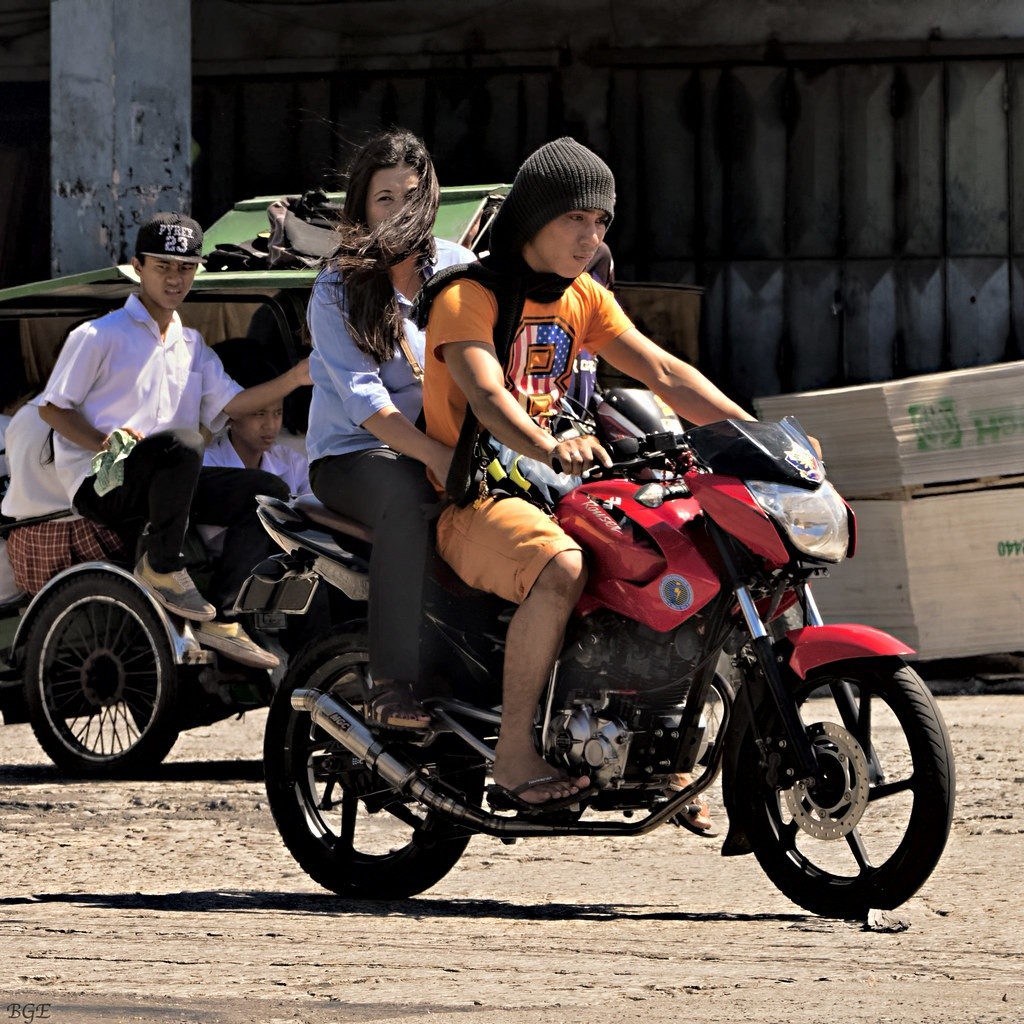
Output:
[471,426,601,509]
[267,188,352,271]
[202,232,272,272]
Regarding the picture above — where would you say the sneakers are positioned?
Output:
[188,619,280,670]
[133,552,216,622]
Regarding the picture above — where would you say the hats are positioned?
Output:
[136,212,208,263]
[486,136,617,260]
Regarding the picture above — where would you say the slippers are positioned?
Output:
[486,769,592,810]
[664,784,720,838]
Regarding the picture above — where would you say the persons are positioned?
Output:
[308,130,482,726]
[1,210,316,669]
[39,211,315,668]
[424,140,826,838]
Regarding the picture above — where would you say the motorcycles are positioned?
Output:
[233,411,956,922]
[0,182,685,772]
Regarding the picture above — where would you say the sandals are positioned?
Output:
[361,674,430,727]
